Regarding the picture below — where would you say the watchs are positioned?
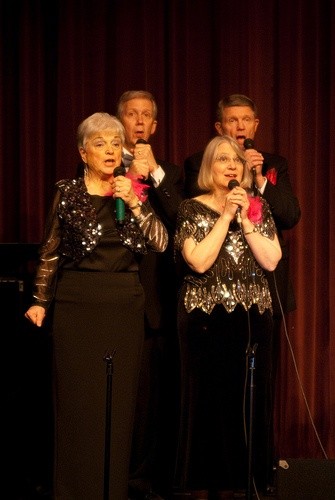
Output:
[130,201,142,210]
[245,228,258,235]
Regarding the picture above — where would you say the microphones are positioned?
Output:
[113,166,126,230]
[136,139,147,144]
[243,138,256,185]
[228,179,242,229]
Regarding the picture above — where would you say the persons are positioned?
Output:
[75,90,184,496]
[174,135,283,500]
[182,95,302,474]
[24,112,169,500]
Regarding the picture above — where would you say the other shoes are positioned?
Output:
[254,470,279,496]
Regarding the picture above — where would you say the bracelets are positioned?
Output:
[125,194,136,203]
